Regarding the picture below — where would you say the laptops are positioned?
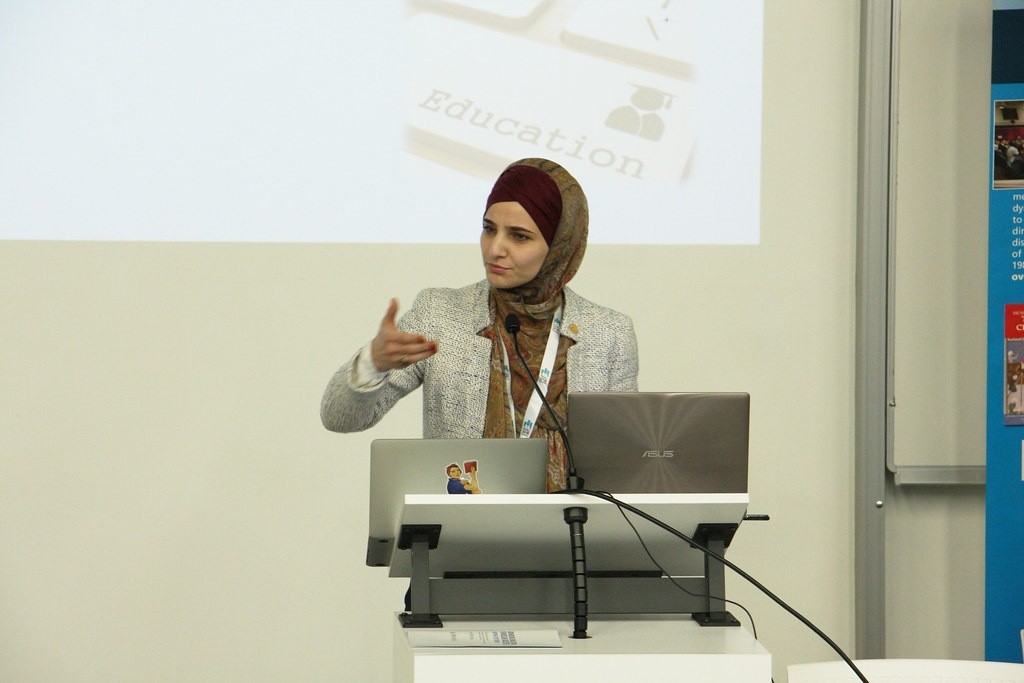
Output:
[367,437,548,566]
[567,390,750,493]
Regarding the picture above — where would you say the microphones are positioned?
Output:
[506,313,584,492]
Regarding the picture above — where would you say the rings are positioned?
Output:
[400,355,409,366]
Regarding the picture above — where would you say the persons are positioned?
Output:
[320,157,639,494]
